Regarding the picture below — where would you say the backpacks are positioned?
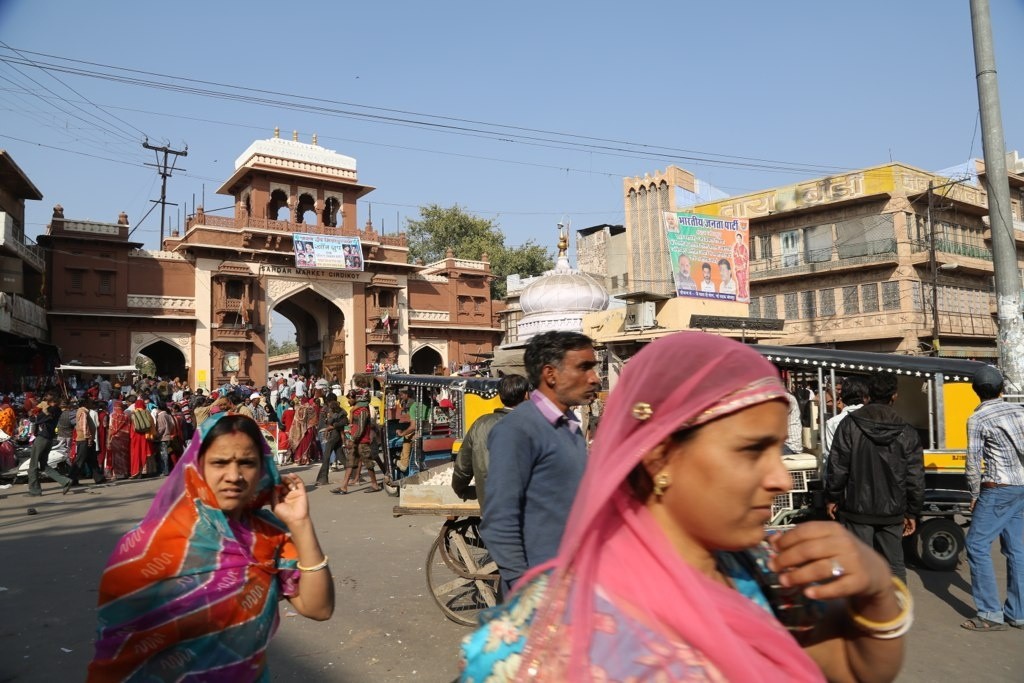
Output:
[132,411,150,432]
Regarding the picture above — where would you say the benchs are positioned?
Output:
[388,434,458,451]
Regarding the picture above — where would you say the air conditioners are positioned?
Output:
[626,301,656,327]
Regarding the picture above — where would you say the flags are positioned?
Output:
[380,311,390,334]
[238,293,249,328]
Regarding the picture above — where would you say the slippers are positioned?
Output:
[364,486,381,494]
[348,480,359,486]
[959,614,1008,630]
[1004,616,1024,630]
[330,487,347,494]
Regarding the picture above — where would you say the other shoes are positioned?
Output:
[62,478,73,495]
[315,481,328,486]
[98,479,110,483]
[128,474,142,480]
[23,490,42,497]
[393,458,407,472]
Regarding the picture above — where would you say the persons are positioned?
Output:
[478,331,601,610]
[678,234,748,298]
[961,369,1024,632]
[827,370,926,586]
[451,374,530,516]
[1,372,455,495]
[454,331,915,683]
[779,376,871,465]
[86,414,336,683]
[570,393,604,444]
[342,245,361,268]
[295,241,315,266]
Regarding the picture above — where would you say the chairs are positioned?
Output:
[781,388,818,470]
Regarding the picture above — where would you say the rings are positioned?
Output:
[831,560,844,577]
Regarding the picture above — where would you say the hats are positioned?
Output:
[250,393,263,400]
[973,367,1003,388]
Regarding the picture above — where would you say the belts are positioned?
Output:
[982,482,1009,488]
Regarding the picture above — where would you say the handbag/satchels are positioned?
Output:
[183,422,193,440]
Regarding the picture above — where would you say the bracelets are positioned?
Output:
[849,577,915,639]
[297,555,329,571]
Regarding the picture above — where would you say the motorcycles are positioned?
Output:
[381,370,538,629]
[744,342,996,576]
